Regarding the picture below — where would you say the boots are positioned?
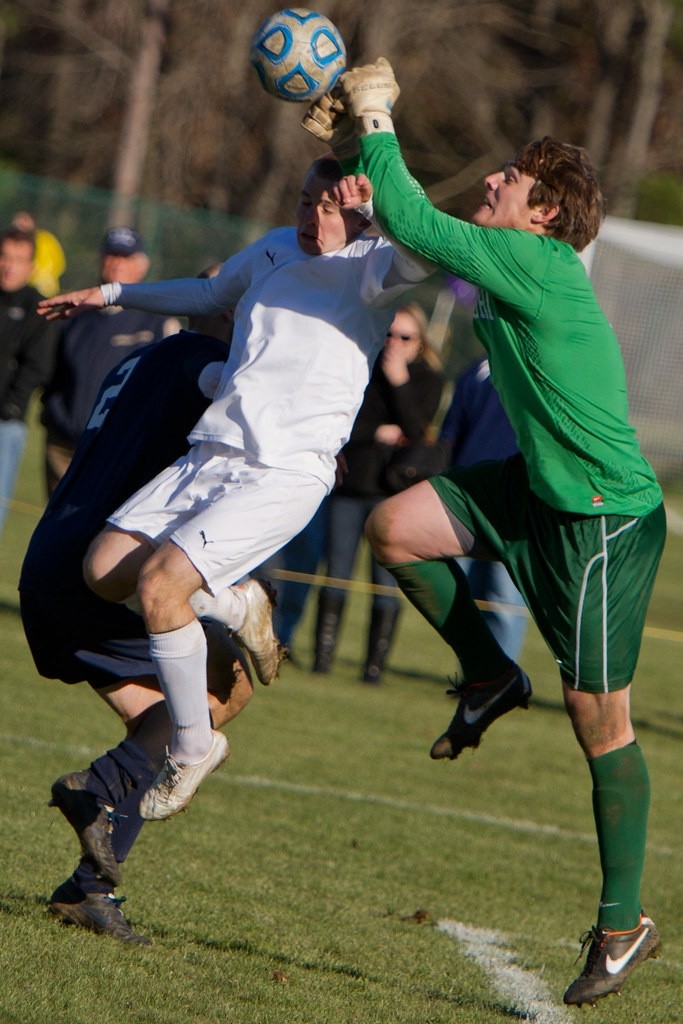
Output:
[365,614,399,687]
[313,605,343,676]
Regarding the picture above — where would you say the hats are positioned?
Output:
[102,224,140,254]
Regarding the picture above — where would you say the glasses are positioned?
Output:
[387,329,418,343]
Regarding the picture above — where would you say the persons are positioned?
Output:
[18,265,252,947]
[1,207,182,521]
[36,158,439,823]
[261,300,526,683]
[303,57,667,1007]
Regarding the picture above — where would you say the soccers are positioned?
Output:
[251,9,348,103]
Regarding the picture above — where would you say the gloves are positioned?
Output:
[301,87,362,157]
[340,56,400,120]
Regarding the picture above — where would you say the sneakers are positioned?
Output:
[46,869,158,947]
[227,578,281,686]
[140,730,231,821]
[562,907,665,1006]
[47,770,123,890]
[429,660,533,762]
